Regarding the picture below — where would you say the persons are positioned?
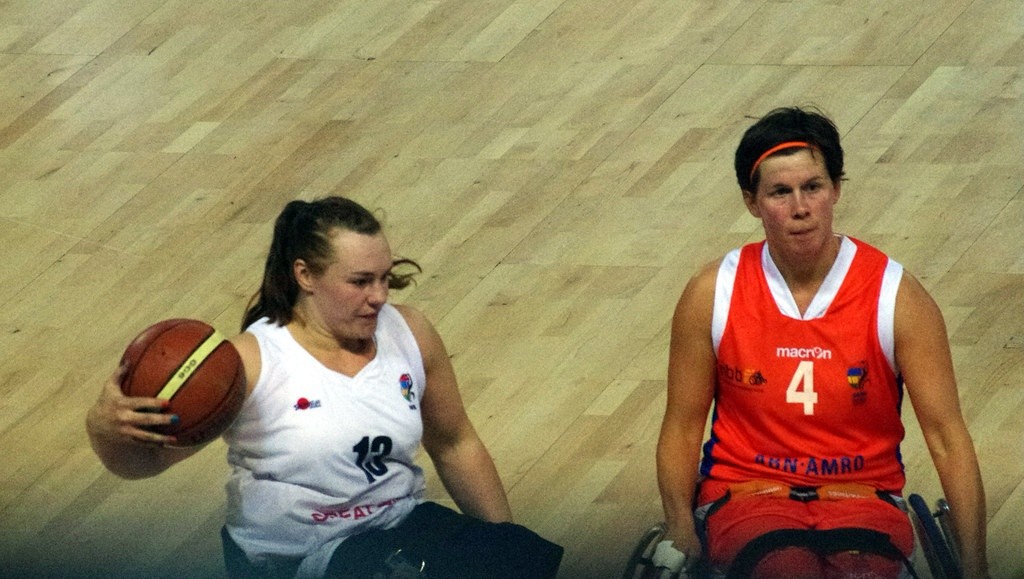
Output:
[647,108,988,579]
[86,197,566,578]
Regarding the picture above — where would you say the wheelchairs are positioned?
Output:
[625,493,968,577]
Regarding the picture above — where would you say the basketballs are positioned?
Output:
[115,316,247,448]
